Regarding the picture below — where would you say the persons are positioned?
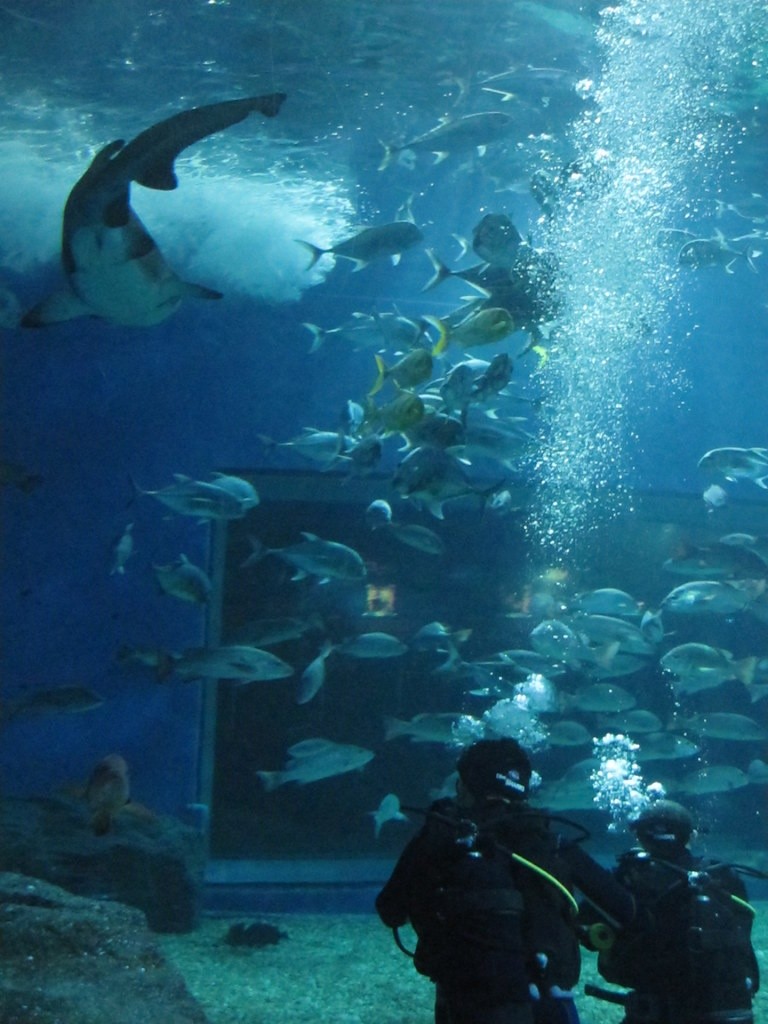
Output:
[374,736,636,1024]
[579,799,760,1024]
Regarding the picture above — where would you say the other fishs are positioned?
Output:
[1,2,767,876]
